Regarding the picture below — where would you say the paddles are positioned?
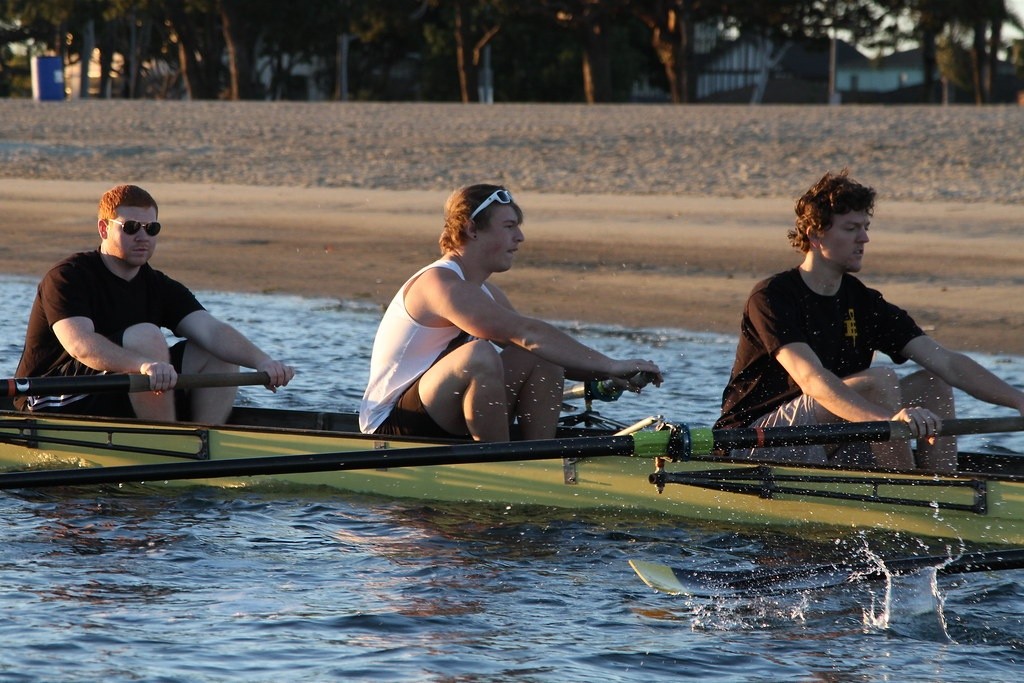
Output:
[1,412,1024,492]
[562,369,655,403]
[0,370,270,399]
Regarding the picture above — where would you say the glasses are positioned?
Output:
[469,190,512,220]
[109,218,161,236]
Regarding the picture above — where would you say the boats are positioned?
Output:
[2,409,1024,554]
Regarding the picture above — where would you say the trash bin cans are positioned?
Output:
[31,56,66,103]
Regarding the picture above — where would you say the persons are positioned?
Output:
[11,184,295,422]
[713,171,1024,470]
[355,184,664,443]
